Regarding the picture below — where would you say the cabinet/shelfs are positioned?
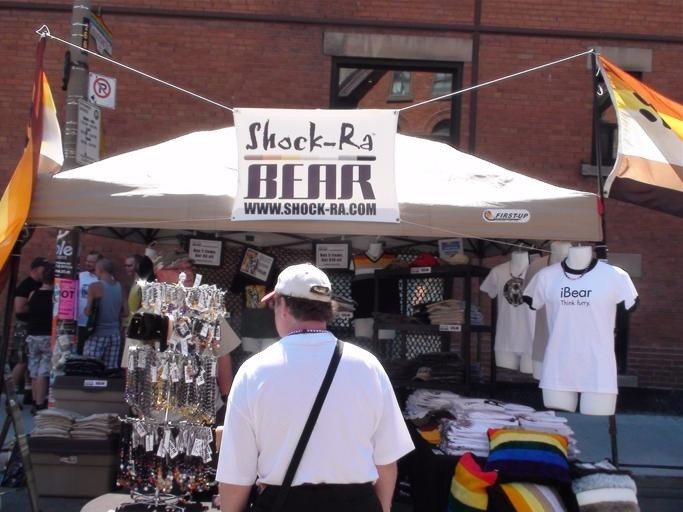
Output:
[372,266,498,399]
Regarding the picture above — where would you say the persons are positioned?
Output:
[526,244,638,417]
[214,263,417,512]
[477,248,534,377]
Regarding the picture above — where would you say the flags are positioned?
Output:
[600,54,683,214]
[0,70,64,263]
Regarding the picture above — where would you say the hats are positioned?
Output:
[145,238,195,274]
[413,367,432,381]
[387,253,469,270]
[260,261,333,304]
[31,256,50,269]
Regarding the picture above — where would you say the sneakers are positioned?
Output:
[14,384,47,415]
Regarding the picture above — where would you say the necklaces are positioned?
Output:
[508,259,527,279]
[561,257,592,280]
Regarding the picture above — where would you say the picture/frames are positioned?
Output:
[240,248,275,283]
[186,238,225,269]
[313,238,352,272]
